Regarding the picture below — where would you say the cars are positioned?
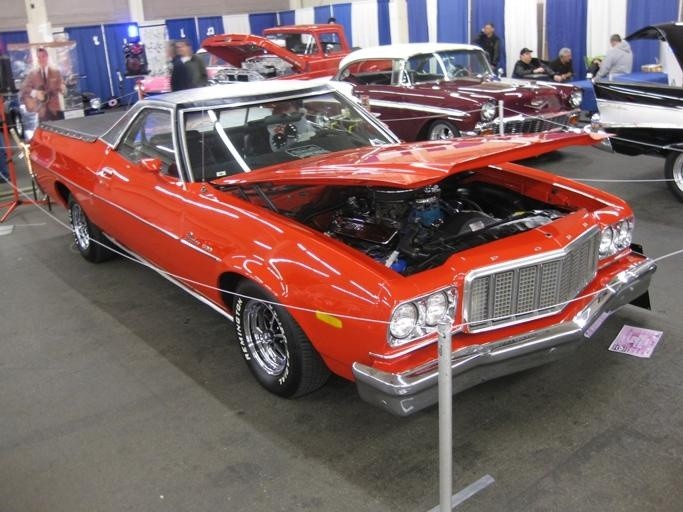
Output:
[585,20,683,203]
[1,77,105,139]
[137,39,284,101]
[324,39,583,159]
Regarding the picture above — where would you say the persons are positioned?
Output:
[473,23,500,76]
[511,48,562,82]
[251,97,302,158]
[22,49,67,125]
[0,134,11,183]
[590,34,633,86]
[165,38,207,93]
[546,48,573,82]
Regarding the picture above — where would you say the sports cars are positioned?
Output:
[27,80,657,416]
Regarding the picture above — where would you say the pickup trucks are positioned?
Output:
[198,22,393,84]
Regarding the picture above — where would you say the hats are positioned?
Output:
[520,48,533,54]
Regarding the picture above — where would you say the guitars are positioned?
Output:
[22,75,87,112]
